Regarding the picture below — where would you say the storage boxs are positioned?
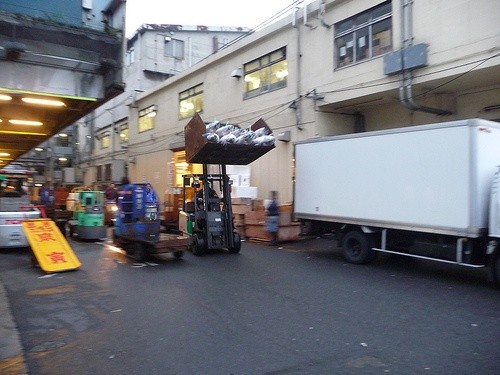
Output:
[231,197,301,245]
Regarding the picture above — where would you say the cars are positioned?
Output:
[0,198,44,250]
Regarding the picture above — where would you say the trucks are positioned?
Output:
[291,118,500,289]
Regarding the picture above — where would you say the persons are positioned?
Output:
[196,180,219,211]
[117,177,135,222]
[264,191,280,246]
[104,183,117,202]
[0,174,68,217]
[145,183,158,206]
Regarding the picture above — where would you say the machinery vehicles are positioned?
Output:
[70,191,107,241]
[179,112,276,256]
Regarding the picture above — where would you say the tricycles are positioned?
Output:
[113,182,192,261]
